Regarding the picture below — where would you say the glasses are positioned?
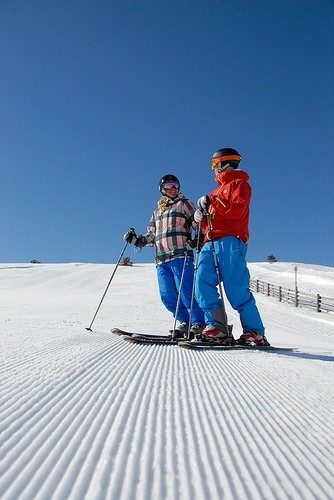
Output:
[212,158,218,170]
[162,180,180,190]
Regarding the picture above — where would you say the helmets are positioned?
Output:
[159,174,180,196]
[213,148,239,170]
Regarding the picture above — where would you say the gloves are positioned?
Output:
[194,209,203,223]
[197,196,209,210]
[124,230,139,248]
[185,240,196,250]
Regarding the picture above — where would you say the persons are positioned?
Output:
[189,148,270,348]
[122,174,208,340]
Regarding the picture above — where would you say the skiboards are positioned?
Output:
[111,328,298,350]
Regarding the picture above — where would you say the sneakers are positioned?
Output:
[187,324,204,340]
[240,331,264,345]
[201,324,227,341]
[169,322,188,337]
